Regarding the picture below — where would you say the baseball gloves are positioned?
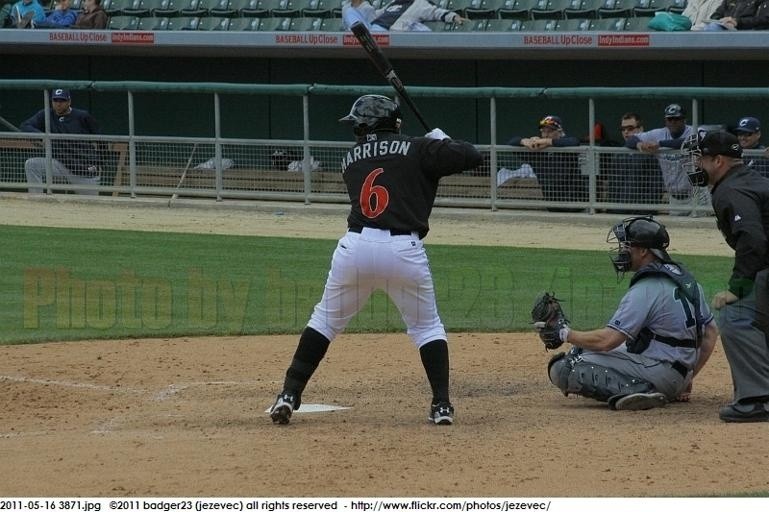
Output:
[531,291,570,352]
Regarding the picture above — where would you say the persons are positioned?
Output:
[536,214,717,409]
[625,104,727,217]
[18,87,107,196]
[268,95,483,425]
[506,116,589,212]
[341,0,464,31]
[680,131,769,422]
[0,0,107,30]
[601,112,667,215]
[681,1,722,30]
[735,118,769,178]
[342,0,375,32]
[701,0,769,31]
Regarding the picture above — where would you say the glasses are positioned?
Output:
[619,126,638,130]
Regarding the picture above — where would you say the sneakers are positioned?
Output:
[270,394,295,424]
[13,6,33,28]
[719,403,769,422]
[615,391,666,410]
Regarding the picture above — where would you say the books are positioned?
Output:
[703,19,736,30]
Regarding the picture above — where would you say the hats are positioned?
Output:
[664,104,682,118]
[736,117,761,133]
[540,116,562,130]
[52,89,70,101]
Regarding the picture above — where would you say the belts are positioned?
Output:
[348,226,411,235]
[672,195,688,200]
[671,360,688,379]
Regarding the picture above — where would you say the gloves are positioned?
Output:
[535,322,571,343]
[424,128,451,141]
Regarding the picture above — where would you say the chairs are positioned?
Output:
[0,0,687,33]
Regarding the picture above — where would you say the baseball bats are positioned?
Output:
[351,22,432,134]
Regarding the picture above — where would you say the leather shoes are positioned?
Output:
[427,401,454,425]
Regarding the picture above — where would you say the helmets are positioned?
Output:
[680,130,744,200]
[338,94,397,126]
[607,215,671,284]
